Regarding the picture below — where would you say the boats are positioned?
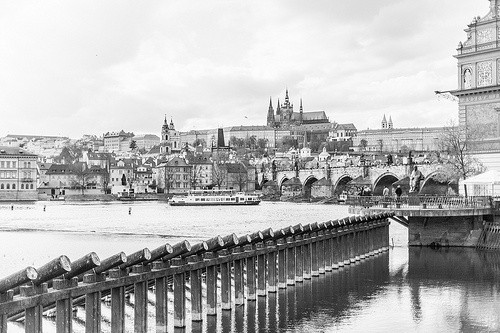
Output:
[168,188,261,206]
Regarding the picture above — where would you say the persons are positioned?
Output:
[382,185,390,202]
[395,185,402,203]
